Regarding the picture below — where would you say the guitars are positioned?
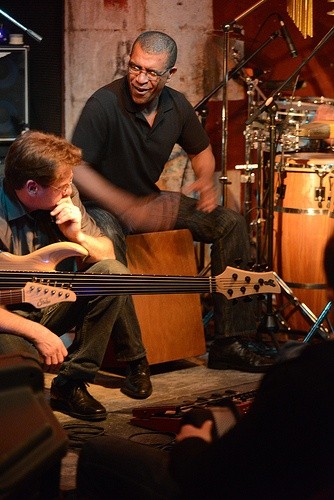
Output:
[0,274,77,311]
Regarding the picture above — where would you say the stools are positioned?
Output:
[101,228,206,368]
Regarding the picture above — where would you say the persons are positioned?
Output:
[67,31,276,399]
[0,129,132,422]
[168,225,334,500]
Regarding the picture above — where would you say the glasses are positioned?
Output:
[126,61,170,81]
[42,178,73,196]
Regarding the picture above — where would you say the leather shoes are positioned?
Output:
[50,377,106,418]
[208,339,277,372]
[123,357,152,397]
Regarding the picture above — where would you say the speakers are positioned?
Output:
[0,354,70,500]
[267,151,334,334]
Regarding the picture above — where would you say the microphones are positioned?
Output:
[278,15,297,59]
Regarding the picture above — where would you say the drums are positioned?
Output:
[0,238,284,302]
[272,152,334,340]
[251,96,334,153]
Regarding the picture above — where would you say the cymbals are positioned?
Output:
[290,119,334,140]
